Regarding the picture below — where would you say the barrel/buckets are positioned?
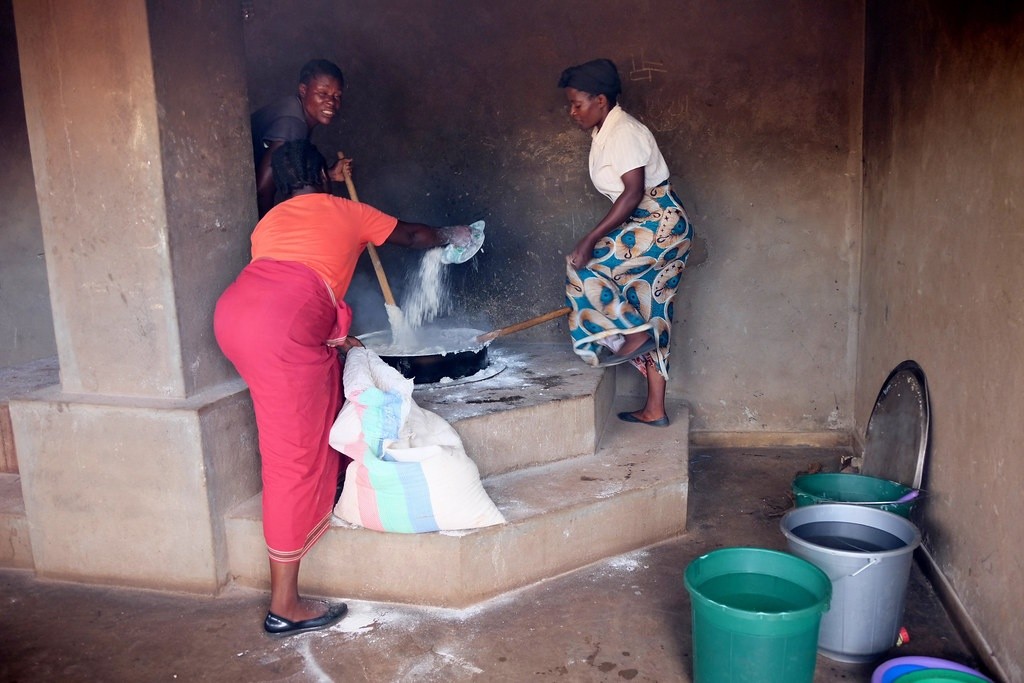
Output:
[682,547,833,683]
[790,472,934,520]
[779,504,922,663]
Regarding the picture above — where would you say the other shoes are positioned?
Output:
[602,338,657,368]
[616,411,669,427]
[263,598,349,637]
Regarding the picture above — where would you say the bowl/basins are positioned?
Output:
[353,324,494,385]
[441,220,486,265]
[871,656,994,683]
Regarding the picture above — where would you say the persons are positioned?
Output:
[558,59,694,427]
[250,59,353,221]
[214,139,481,635]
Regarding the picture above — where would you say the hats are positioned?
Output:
[559,58,622,99]
